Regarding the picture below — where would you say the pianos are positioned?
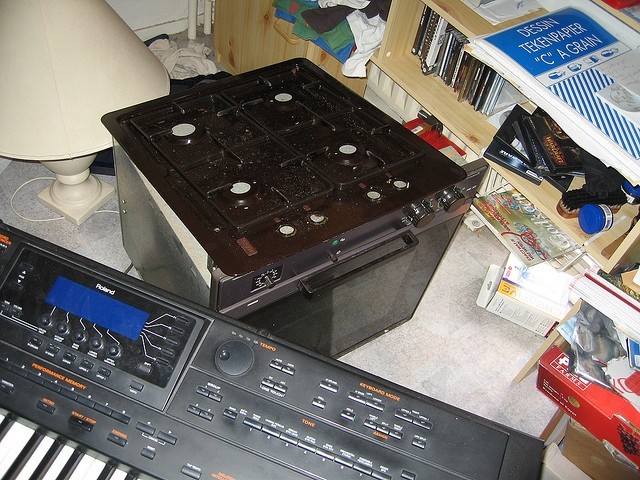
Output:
[0,223,544,479]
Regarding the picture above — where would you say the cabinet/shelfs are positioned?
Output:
[371,0,639,274]
[213,0,371,98]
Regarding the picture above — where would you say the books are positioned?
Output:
[410,4,506,117]
[463,5,639,188]
[470,190,580,267]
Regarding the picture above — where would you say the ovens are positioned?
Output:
[99,56,489,358]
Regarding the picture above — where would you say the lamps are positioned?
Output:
[0,0,172,228]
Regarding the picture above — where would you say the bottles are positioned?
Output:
[578,204,613,235]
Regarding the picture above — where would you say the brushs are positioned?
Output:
[556,186,628,219]
